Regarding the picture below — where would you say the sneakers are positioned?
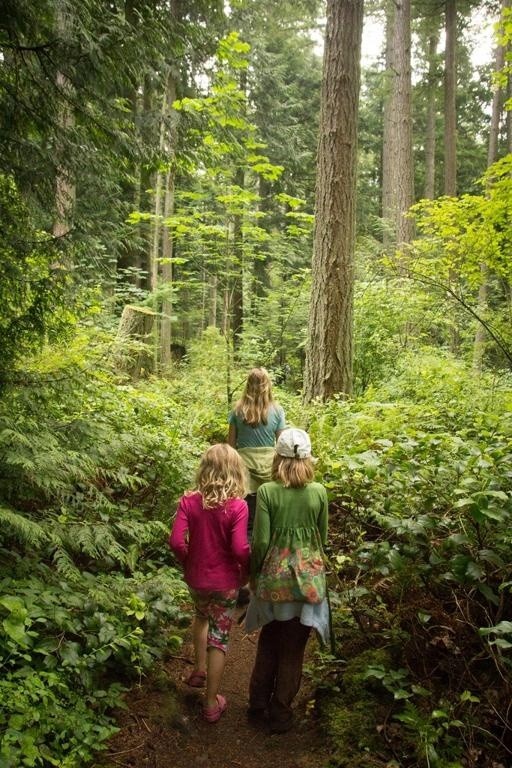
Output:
[247,700,296,734]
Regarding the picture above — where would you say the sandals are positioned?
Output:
[186,670,208,688]
[202,694,226,723]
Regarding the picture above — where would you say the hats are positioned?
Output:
[275,428,312,458]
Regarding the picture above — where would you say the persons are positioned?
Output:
[169,443,251,724]
[249,428,329,734]
[228,369,285,545]
